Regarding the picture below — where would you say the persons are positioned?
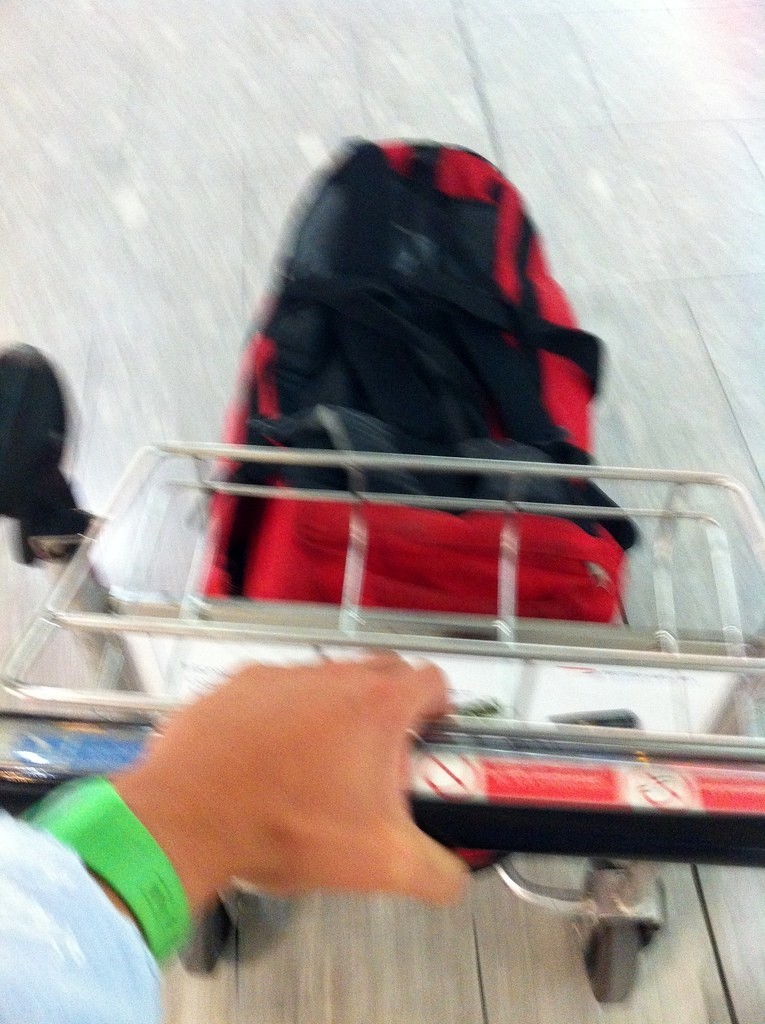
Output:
[3,641,472,1024]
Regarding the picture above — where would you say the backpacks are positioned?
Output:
[205,142,636,620]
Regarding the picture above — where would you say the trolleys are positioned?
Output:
[0,433,765,1007]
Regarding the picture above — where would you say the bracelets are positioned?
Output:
[22,774,196,964]
